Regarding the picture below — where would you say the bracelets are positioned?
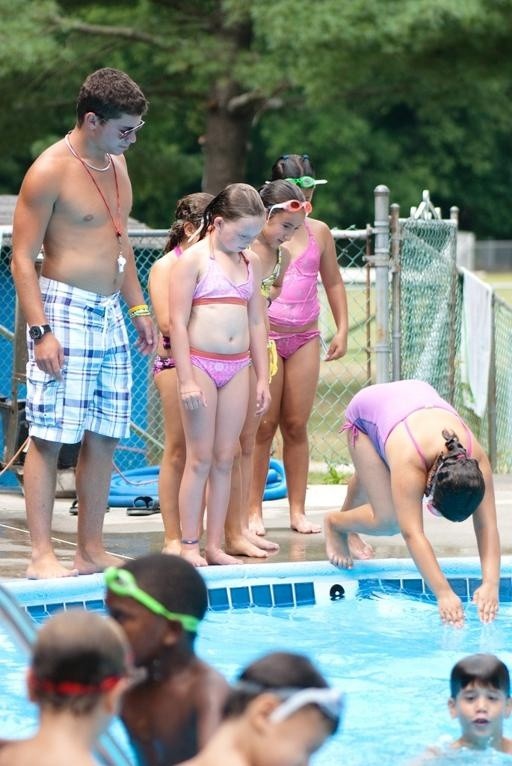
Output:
[128,304,151,319]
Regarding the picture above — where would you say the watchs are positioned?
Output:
[30,324,50,339]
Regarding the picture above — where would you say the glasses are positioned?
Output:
[102,563,201,631]
[96,117,145,141]
[272,200,313,213]
[284,175,317,189]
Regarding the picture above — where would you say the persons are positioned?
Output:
[10,67,158,580]
[159,652,349,766]
[217,180,312,560]
[420,654,511,766]
[105,554,230,766]
[168,183,272,567]
[1,609,130,766]
[251,155,349,534]
[323,376,501,628]
[152,193,218,556]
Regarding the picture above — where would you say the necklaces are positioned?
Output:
[64,133,112,171]
